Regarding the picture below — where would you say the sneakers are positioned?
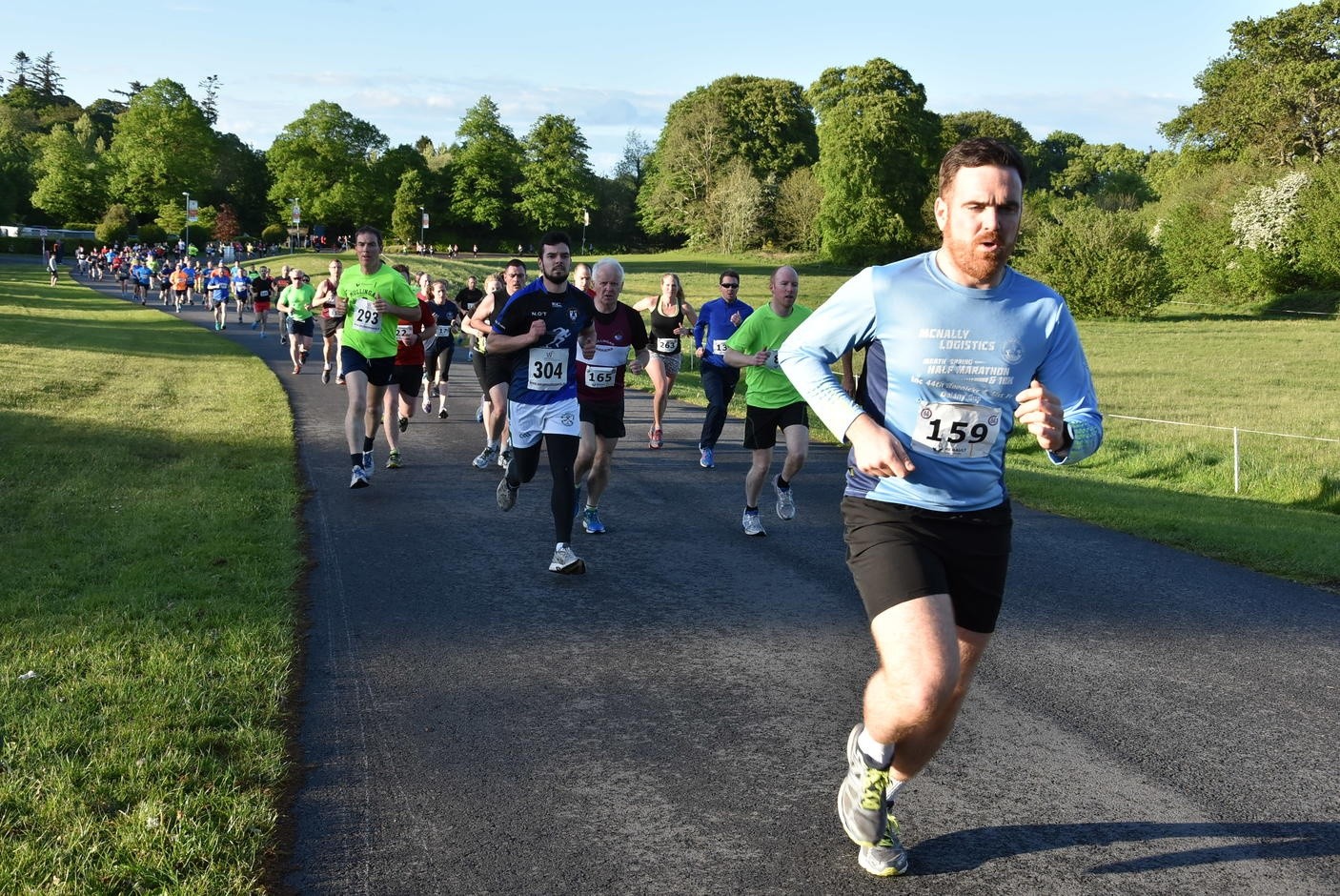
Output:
[472,446,498,469]
[498,452,512,468]
[213,316,347,386]
[548,544,586,575]
[742,507,766,536]
[837,722,894,847]
[363,447,374,478]
[699,442,715,468]
[386,449,403,467]
[495,460,518,511]
[476,395,483,423]
[583,508,605,534]
[773,473,795,520]
[648,425,663,449]
[857,800,908,877]
[350,465,369,488]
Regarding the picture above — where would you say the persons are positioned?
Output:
[169,264,188,312]
[723,265,817,535]
[486,229,598,574]
[136,261,154,306]
[630,273,698,451]
[574,258,650,535]
[381,264,438,468]
[776,134,1103,877]
[276,269,317,375]
[340,228,423,489]
[47,227,599,474]
[694,272,754,471]
[207,267,230,331]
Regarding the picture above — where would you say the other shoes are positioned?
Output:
[438,408,448,419]
[398,417,407,431]
[421,401,431,413]
[121,291,214,313]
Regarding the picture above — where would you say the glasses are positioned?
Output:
[293,277,302,280]
[302,279,308,282]
[722,283,738,288]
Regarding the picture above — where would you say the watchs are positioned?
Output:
[1047,419,1076,455]
[687,328,694,337]
